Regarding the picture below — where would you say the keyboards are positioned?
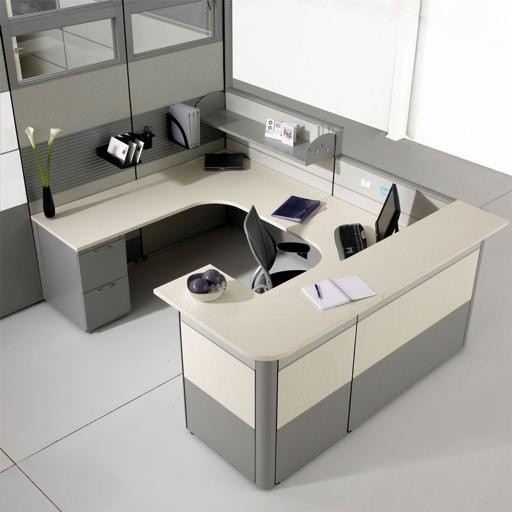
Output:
[335,222,367,261]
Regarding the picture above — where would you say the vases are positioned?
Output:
[42,188,55,219]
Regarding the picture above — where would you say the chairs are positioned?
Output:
[243,205,312,295]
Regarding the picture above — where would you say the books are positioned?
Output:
[170,102,202,150]
[301,275,378,310]
[270,194,320,222]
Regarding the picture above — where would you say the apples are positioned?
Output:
[190,268,222,294]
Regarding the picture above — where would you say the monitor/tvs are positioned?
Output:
[374,182,401,243]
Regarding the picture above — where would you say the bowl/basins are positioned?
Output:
[187,272,227,303]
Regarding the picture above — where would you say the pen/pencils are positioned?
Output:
[315,283,321,298]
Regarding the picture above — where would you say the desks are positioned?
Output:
[29,148,407,492]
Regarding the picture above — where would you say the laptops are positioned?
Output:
[204,152,245,171]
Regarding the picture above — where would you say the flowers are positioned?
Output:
[24,126,62,187]
